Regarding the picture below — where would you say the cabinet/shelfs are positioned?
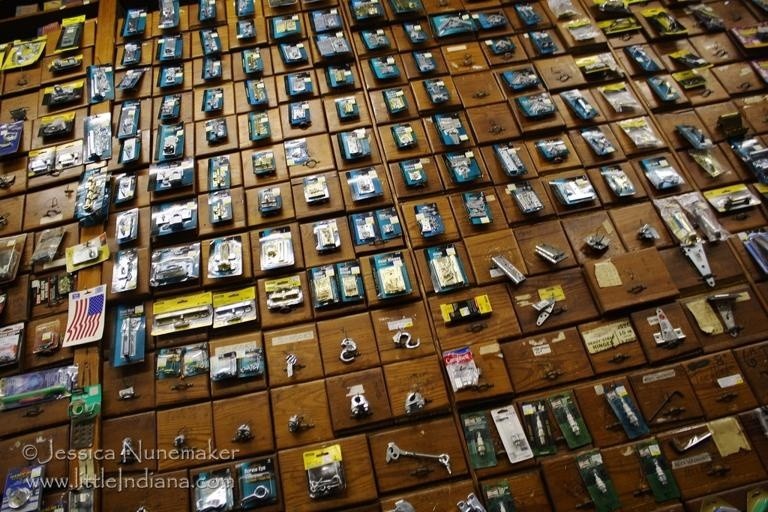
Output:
[0,0,768,512]
